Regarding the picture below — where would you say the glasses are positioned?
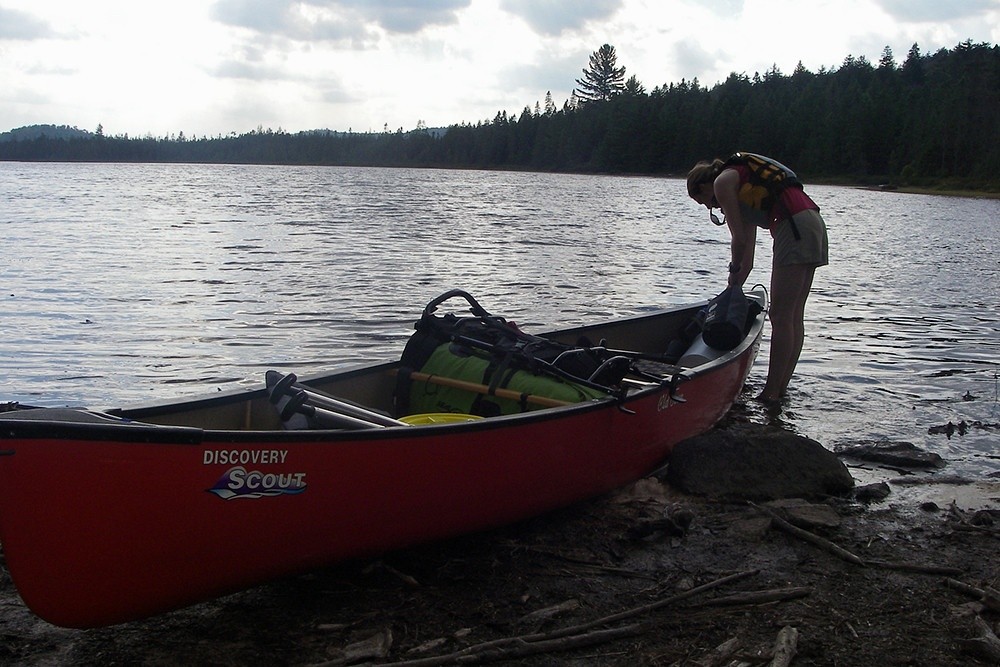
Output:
[709,206,726,225]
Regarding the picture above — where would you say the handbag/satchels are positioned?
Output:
[702,283,763,352]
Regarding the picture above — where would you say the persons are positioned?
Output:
[687,152,829,404]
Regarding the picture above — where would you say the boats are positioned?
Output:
[0,283,768,630]
